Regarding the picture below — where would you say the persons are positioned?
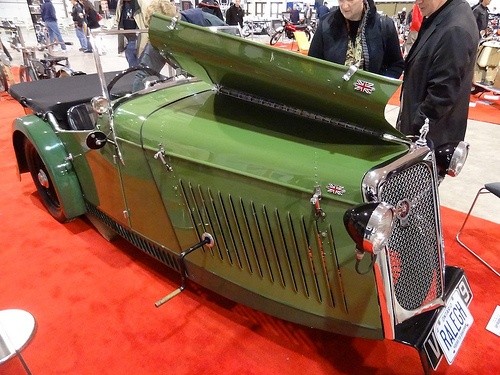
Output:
[319,2,330,16]
[115,0,141,68]
[308,0,405,79]
[472,0,492,38]
[409,0,423,32]
[395,0,479,150]
[407,9,413,26]
[70,0,101,53]
[400,11,406,24]
[226,0,243,28]
[41,0,66,50]
[290,6,299,23]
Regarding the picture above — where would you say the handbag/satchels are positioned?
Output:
[95,12,103,21]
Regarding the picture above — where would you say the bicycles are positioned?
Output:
[9,40,75,83]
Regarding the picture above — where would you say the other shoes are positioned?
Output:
[80,48,86,50]
[63,49,68,52]
[85,51,92,53]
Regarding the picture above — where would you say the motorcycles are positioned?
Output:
[269,15,311,45]
